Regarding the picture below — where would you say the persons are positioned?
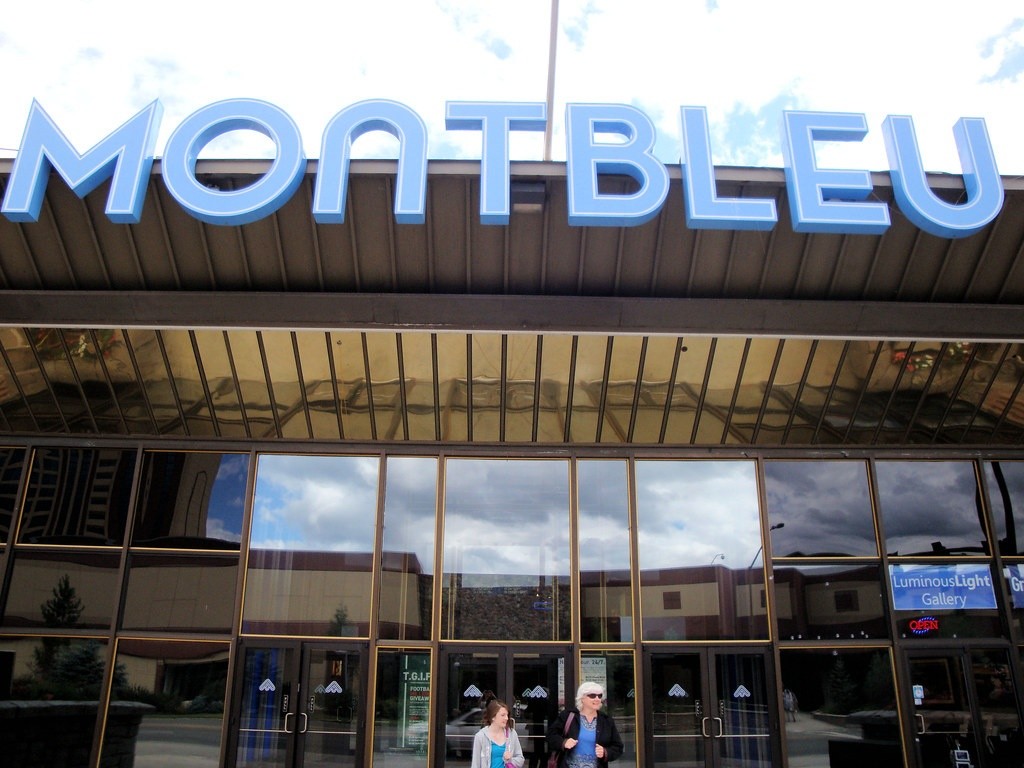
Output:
[471,701,525,768]
[545,681,624,768]
[781,688,800,723]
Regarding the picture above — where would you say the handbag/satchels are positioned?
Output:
[548,751,557,768]
[505,763,516,768]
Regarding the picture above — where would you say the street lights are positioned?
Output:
[745,522,786,639]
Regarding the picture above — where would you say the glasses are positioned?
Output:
[587,693,602,699]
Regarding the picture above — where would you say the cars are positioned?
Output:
[408,704,548,759]
